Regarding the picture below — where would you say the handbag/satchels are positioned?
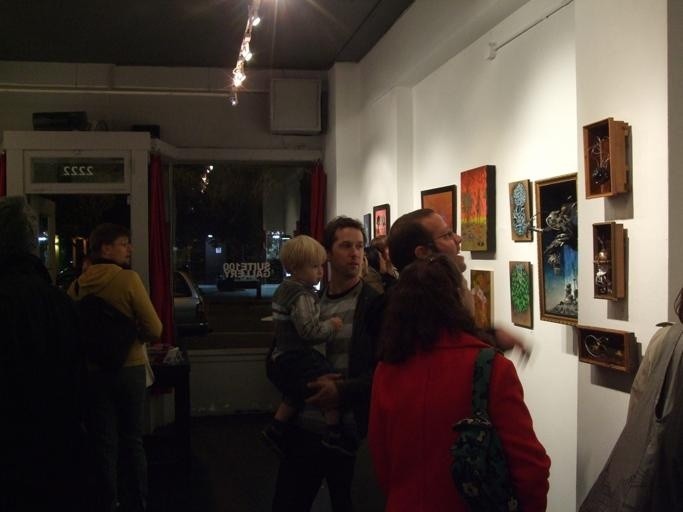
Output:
[447,413,520,512]
[76,294,135,381]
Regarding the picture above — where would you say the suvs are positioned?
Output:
[172,271,204,327]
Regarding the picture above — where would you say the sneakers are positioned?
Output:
[322,430,353,456]
[258,425,286,458]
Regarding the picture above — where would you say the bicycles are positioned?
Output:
[585,334,623,365]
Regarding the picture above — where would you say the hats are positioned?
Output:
[0,193,38,256]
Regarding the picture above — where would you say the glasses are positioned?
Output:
[113,239,129,249]
[425,254,456,291]
[422,231,453,246]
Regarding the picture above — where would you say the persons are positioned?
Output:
[368,209,526,405]
[0,195,52,512]
[256,233,357,462]
[273,216,378,512]
[367,252,549,511]
[361,234,400,295]
[577,287,682,511]
[64,222,164,511]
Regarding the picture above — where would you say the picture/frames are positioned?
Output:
[373,204,390,238]
[421,185,457,234]
[535,173,578,326]
[364,213,371,248]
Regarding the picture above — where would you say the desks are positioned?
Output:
[148,344,190,495]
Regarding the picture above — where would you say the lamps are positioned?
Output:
[234,10,261,90]
